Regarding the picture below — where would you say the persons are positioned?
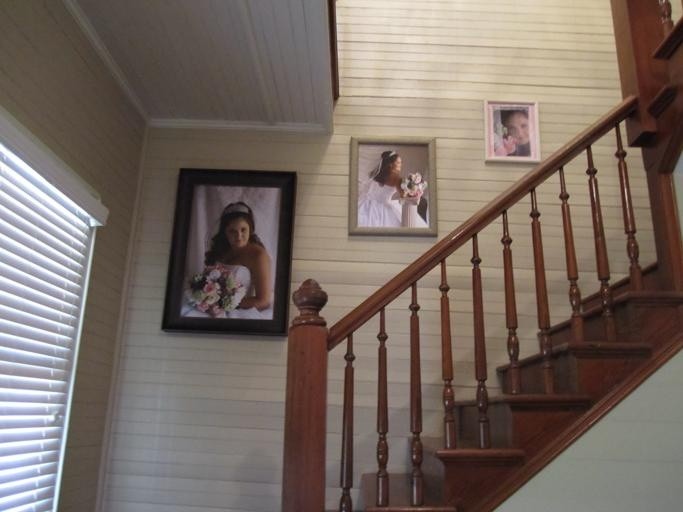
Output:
[356,149,425,227]
[492,110,532,158]
[182,199,273,320]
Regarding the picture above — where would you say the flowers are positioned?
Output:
[494,121,516,155]
[400,172,428,198]
[186,263,248,317]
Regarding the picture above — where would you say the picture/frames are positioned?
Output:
[485,100,541,162]
[161,167,298,335]
[347,137,438,236]
